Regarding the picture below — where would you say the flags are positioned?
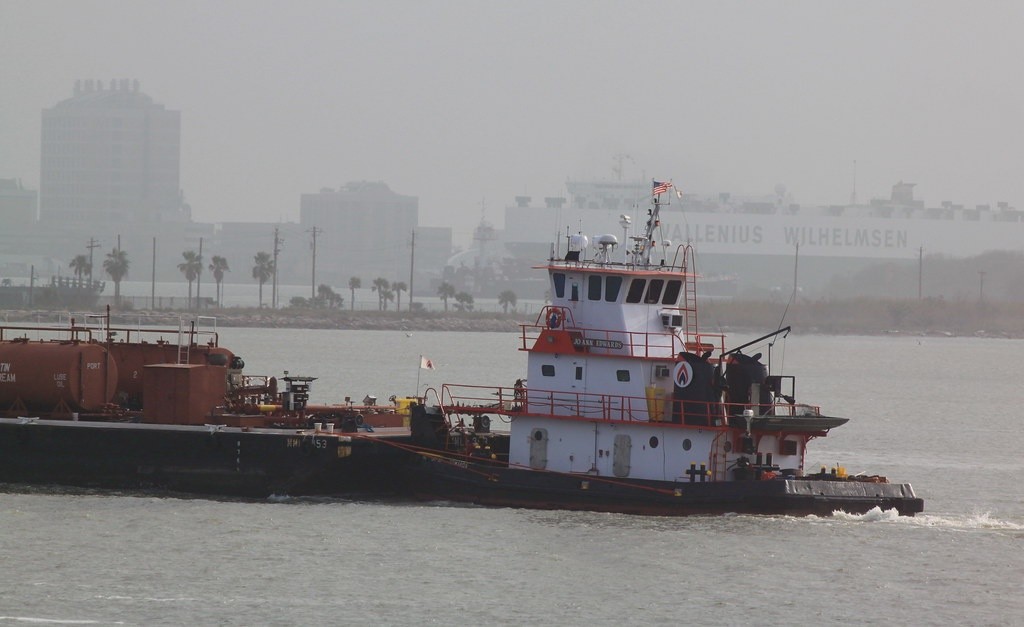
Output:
[653,181,672,195]
[676,191,684,199]
[421,356,435,370]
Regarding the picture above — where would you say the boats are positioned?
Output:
[0,180,924,519]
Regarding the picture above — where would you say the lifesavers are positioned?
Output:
[545,307,562,328]
